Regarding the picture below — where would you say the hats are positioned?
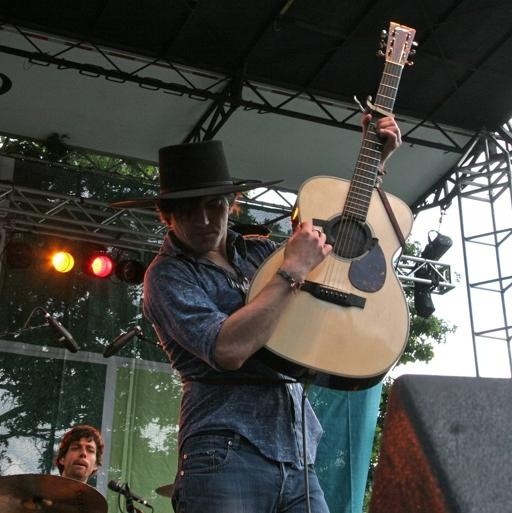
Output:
[107,140,284,207]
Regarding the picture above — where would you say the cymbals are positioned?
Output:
[0,474,108,512]
[155,483,174,497]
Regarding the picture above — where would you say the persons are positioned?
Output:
[55,424,103,482]
[141,107,404,512]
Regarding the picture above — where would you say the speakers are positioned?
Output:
[368,373,511,512]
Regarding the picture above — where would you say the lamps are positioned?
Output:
[0,230,148,288]
[412,281,436,319]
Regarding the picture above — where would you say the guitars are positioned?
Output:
[245,22,418,391]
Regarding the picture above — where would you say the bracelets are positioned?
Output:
[374,169,385,183]
[277,266,305,295]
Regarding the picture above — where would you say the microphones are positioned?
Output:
[108,480,148,507]
[104,325,142,358]
[45,312,79,353]
